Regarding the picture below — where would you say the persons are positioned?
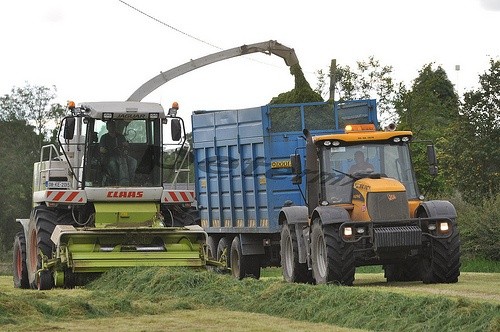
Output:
[350,150,376,174]
[99,120,138,185]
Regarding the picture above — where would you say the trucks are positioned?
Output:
[191,99,460,284]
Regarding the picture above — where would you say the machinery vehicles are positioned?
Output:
[11,42,300,290]
